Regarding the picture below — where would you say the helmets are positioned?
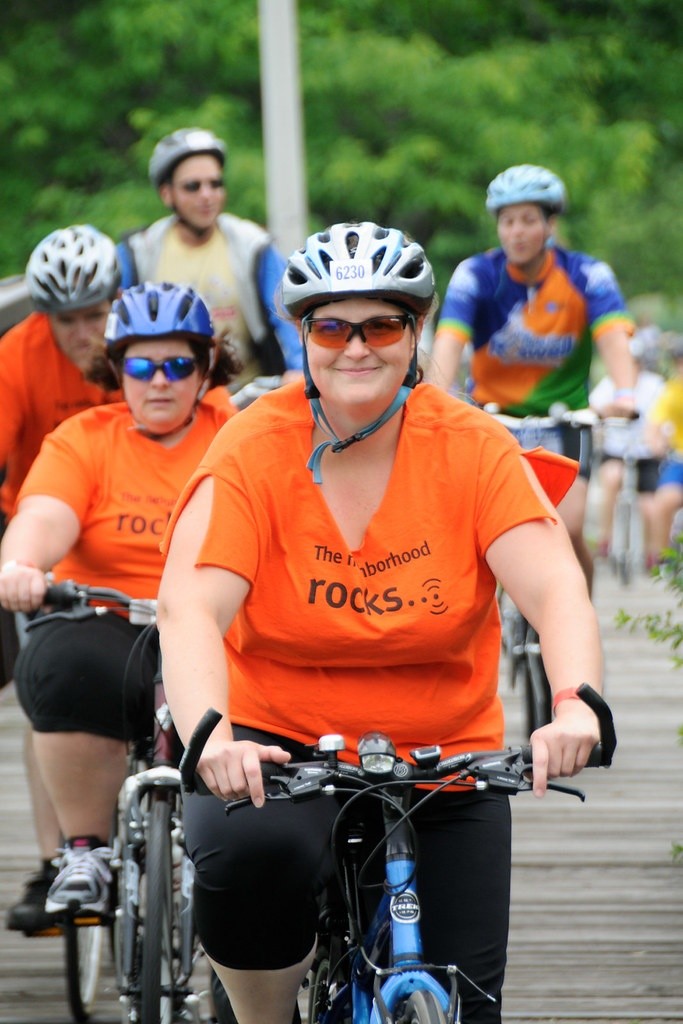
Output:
[278,221,440,321]
[103,279,216,360]
[146,126,229,187]
[485,163,565,218]
[25,224,122,313]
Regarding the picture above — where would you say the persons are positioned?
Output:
[0,226,126,927]
[0,280,240,924]
[428,162,640,602]
[116,129,302,405]
[590,324,683,576]
[158,222,604,1023]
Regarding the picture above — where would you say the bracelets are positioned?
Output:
[4,560,33,569]
[553,686,580,710]
[614,389,630,397]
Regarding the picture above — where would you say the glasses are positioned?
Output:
[115,356,201,382]
[168,176,225,193]
[301,314,412,350]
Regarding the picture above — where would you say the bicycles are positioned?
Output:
[179,684,618,1023]
[11,577,198,1024]
[452,398,641,747]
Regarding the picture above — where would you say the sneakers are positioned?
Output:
[44,835,124,914]
[7,861,63,932]
